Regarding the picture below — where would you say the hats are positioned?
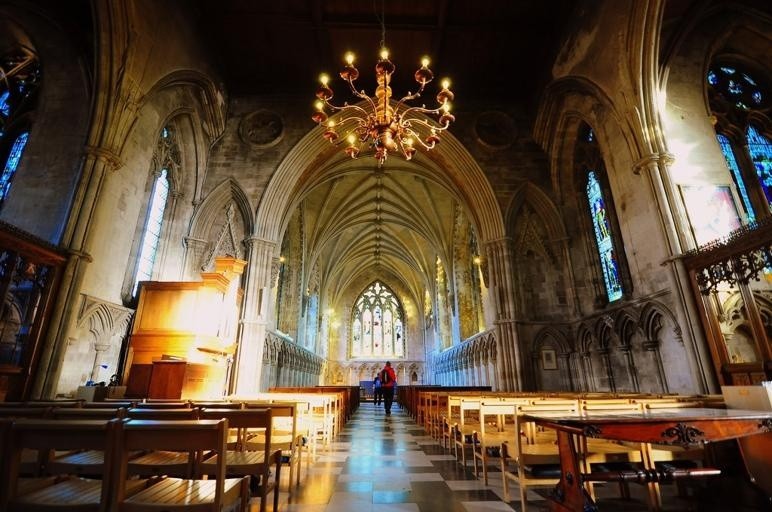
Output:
[386,361,391,364]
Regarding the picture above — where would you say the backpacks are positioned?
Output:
[381,371,391,385]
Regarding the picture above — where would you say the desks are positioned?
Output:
[527,410,772,512]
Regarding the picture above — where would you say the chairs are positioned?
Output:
[500,400,583,510]
[124,406,199,478]
[193,406,283,510]
[470,402,516,487]
[584,398,658,510]
[450,396,480,466]
[243,401,305,498]
[109,407,251,512]
[45,408,127,474]
[8,412,108,512]
[645,400,708,512]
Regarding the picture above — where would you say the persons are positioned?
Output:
[382,360,396,417]
[375,373,382,406]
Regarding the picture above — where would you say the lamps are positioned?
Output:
[310,0,457,168]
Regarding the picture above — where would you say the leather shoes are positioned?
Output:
[386,410,390,413]
